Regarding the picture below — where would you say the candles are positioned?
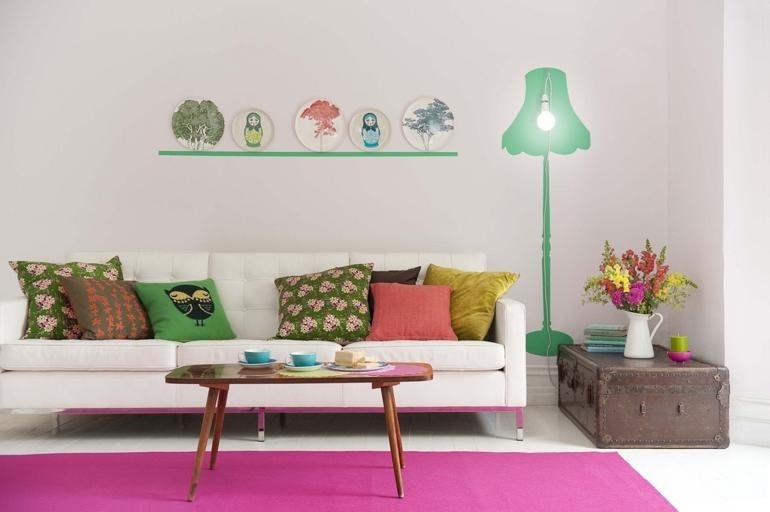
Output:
[669,332,688,352]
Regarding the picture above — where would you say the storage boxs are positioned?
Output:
[555,345,730,450]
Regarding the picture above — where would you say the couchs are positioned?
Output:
[1,248,528,440]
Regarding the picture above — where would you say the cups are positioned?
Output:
[237,348,271,364]
[284,350,316,366]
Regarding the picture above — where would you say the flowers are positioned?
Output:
[580,236,704,314]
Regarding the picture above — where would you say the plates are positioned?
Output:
[231,107,275,149]
[282,360,322,371]
[325,360,391,370]
[400,94,455,150]
[236,367,276,376]
[237,358,277,370]
[348,107,392,150]
[171,95,226,150]
[293,97,346,150]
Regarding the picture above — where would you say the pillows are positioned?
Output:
[269,261,377,349]
[56,272,152,340]
[365,279,459,346]
[133,275,236,341]
[7,257,122,340]
[422,258,521,342]
[362,261,424,327]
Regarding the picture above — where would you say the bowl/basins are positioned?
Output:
[667,350,693,363]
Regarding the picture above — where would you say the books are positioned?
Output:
[581,323,626,353]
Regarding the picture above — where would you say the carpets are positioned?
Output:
[0,448,680,512]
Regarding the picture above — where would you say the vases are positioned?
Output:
[624,309,665,359]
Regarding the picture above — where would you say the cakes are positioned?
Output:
[335,350,365,368]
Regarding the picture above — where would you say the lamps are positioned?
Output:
[502,65,591,356]
